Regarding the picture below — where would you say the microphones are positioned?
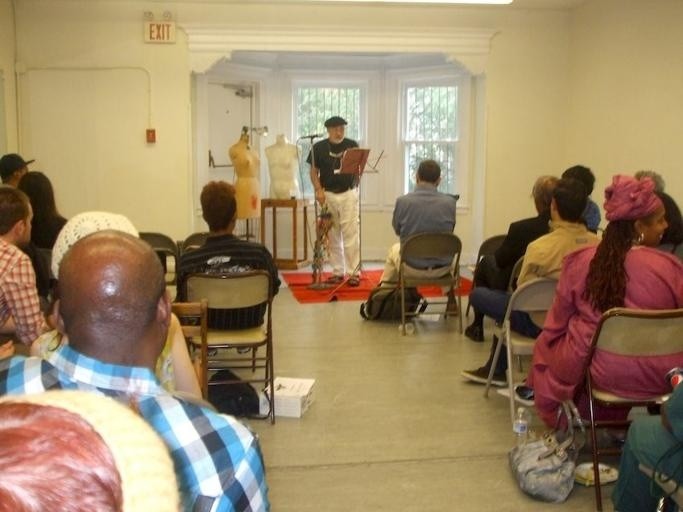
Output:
[300,133,324,139]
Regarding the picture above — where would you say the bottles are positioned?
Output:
[512,407,527,446]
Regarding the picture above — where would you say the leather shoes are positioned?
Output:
[465,322,483,341]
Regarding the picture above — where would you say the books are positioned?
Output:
[335,147,371,176]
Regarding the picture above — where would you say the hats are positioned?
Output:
[0,154,35,176]
[325,117,347,126]
[604,175,662,221]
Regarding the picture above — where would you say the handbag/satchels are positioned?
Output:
[507,399,587,504]
[207,370,260,416]
[360,287,428,319]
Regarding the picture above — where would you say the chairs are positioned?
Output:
[35,233,275,399]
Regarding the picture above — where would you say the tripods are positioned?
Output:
[326,164,376,298]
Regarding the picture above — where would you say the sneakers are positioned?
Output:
[462,367,506,387]
[349,275,359,285]
[329,276,343,280]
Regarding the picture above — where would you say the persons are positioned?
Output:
[305,117,364,288]
[609,386,683,510]
[227,133,261,222]
[1,150,284,511]
[262,134,301,199]
[460,161,683,464]
[377,160,460,317]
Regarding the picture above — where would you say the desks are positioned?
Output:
[261,198,314,269]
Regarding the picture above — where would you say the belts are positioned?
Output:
[425,266,443,270]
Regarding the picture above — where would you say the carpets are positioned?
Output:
[282,270,473,304]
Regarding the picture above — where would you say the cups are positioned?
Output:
[290,190,295,200]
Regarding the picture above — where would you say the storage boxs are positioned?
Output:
[259,376,317,418]
